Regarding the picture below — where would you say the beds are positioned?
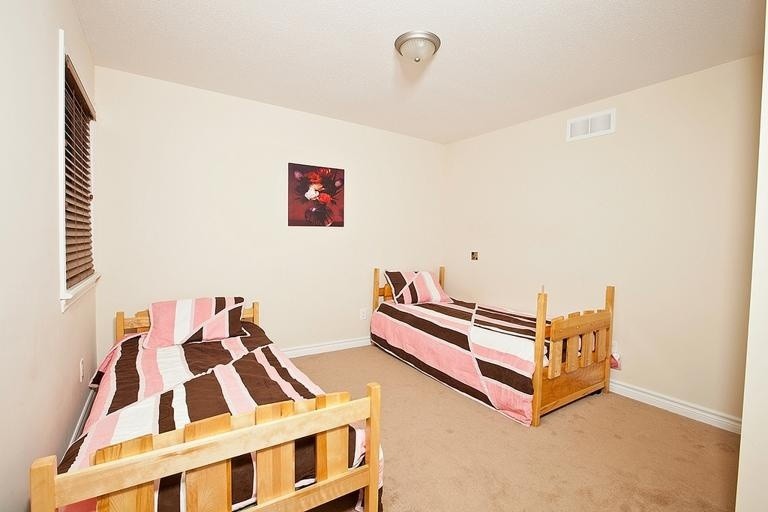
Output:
[371,267,615,429]
[31,301,383,511]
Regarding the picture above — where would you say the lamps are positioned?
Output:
[395,30,441,67]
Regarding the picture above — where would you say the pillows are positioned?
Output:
[384,269,455,308]
[145,296,248,348]
